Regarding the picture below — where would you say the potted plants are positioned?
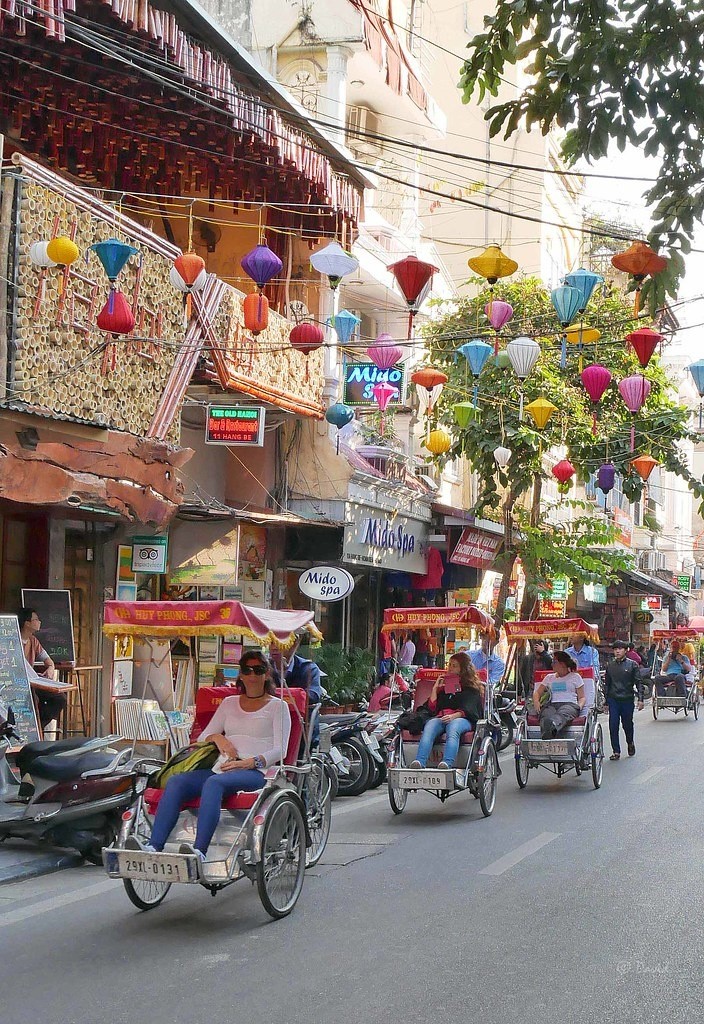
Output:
[296,642,379,717]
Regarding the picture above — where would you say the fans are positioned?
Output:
[187,219,221,252]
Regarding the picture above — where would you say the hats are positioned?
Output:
[239,650,268,666]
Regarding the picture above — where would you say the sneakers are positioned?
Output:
[125,835,158,852]
[179,843,206,862]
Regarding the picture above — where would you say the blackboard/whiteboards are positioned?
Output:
[21,589,76,668]
[0,615,42,753]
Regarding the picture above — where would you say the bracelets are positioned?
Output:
[254,755,264,768]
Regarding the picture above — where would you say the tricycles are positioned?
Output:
[376,604,499,818]
[82,601,335,919]
[503,618,606,790]
[650,628,701,720]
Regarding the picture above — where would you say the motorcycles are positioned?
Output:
[297,681,398,797]
[594,665,655,714]
[494,692,520,752]
[0,682,148,873]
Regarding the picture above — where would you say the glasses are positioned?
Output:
[241,665,267,676]
[552,660,566,665]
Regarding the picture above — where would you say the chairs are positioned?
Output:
[660,658,696,688]
[401,668,489,745]
[142,684,309,816]
[526,667,598,725]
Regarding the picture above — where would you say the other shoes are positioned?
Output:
[610,754,620,760]
[409,760,422,769]
[628,745,635,756]
[540,717,552,734]
[437,762,449,769]
[542,726,557,740]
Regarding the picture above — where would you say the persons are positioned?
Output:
[533,651,586,739]
[462,628,506,776]
[603,640,645,760]
[626,642,656,668]
[367,672,398,711]
[125,651,291,869]
[564,634,600,682]
[655,641,691,698]
[520,639,552,699]
[225,636,321,867]
[408,651,482,767]
[17,608,67,731]
[679,637,695,685]
[397,633,416,665]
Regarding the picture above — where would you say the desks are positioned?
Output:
[32,662,102,740]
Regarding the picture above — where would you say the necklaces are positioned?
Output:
[245,690,265,699]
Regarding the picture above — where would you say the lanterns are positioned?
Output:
[28,229,704,493]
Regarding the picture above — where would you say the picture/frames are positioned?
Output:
[104,518,271,764]
